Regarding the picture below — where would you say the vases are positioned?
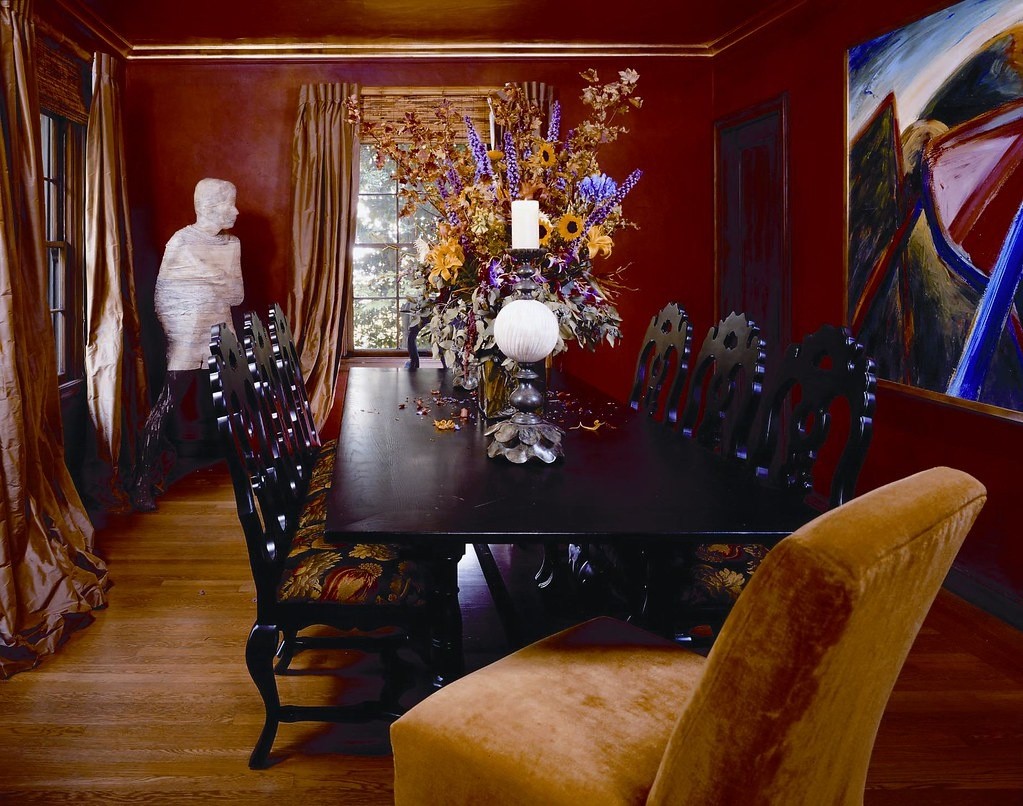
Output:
[471,357,550,419]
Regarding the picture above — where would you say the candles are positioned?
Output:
[511,196,540,249]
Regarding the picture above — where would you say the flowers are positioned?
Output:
[342,66,645,388]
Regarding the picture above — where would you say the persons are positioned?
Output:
[125,177,245,512]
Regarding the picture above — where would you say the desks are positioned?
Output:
[322,366,824,653]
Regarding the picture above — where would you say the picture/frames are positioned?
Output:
[840,0,1023,422]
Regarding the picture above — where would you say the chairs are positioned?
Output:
[388,466,989,805]
[206,301,465,771]
[568,301,878,648]
